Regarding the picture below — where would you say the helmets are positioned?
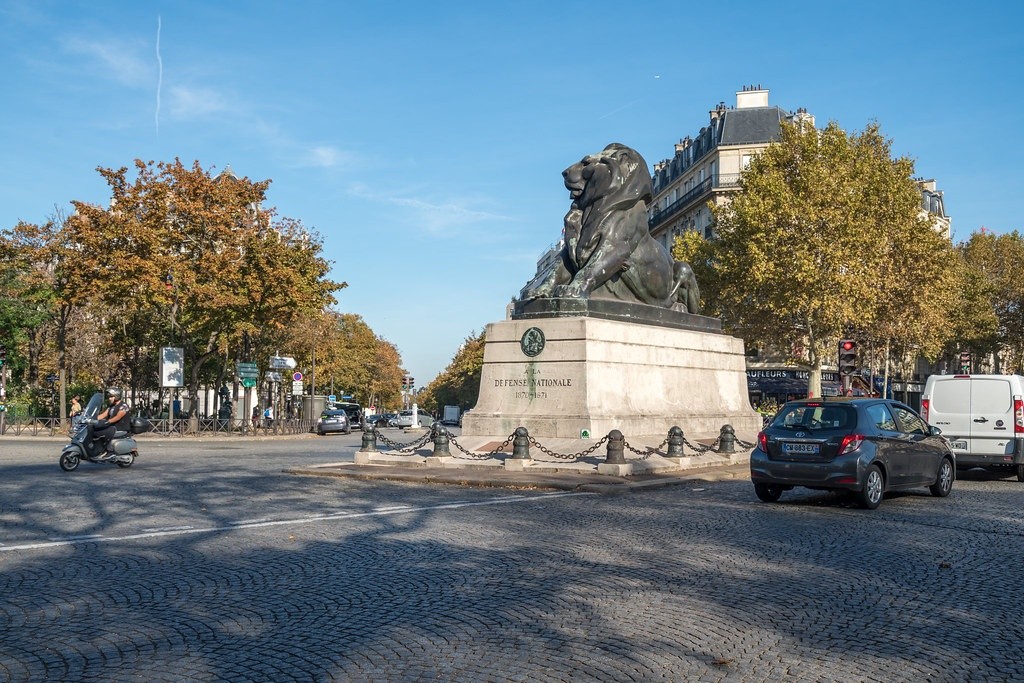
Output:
[105,386,123,402]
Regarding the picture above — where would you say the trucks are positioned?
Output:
[443,405,461,426]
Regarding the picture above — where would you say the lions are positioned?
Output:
[519,141,702,313]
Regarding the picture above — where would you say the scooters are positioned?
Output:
[59,392,151,472]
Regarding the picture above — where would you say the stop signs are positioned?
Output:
[370,406,376,411]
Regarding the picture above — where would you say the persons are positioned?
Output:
[92,386,131,459]
[69,395,86,429]
[252,404,297,429]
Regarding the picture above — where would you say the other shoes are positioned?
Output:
[93,450,107,458]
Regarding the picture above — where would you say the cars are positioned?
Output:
[367,414,398,429]
[398,408,435,430]
[317,409,352,435]
[749,397,958,511]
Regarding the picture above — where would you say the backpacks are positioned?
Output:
[264,409,269,417]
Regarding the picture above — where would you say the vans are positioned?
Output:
[922,372,1024,483]
[333,402,364,429]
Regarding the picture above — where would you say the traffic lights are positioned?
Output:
[838,339,857,375]
[409,376,415,389]
[402,377,408,390]
